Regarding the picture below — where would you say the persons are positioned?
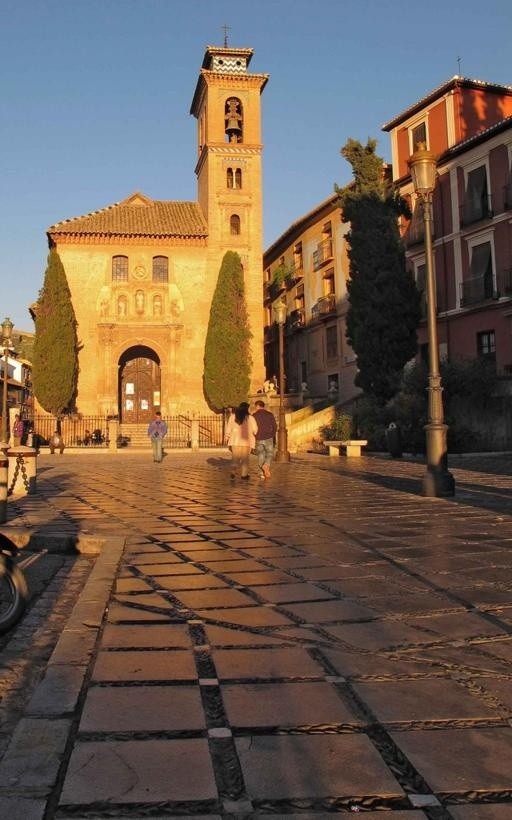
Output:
[71,428,103,446]
[49,431,65,454]
[251,400,277,478]
[147,411,168,464]
[224,402,258,481]
[12,414,24,447]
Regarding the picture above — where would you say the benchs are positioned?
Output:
[322,440,368,458]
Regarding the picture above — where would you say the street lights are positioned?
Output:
[1,317,15,443]
[273,297,290,465]
[404,139,456,496]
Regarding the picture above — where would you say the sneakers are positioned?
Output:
[261,463,271,479]
[230,474,250,481]
